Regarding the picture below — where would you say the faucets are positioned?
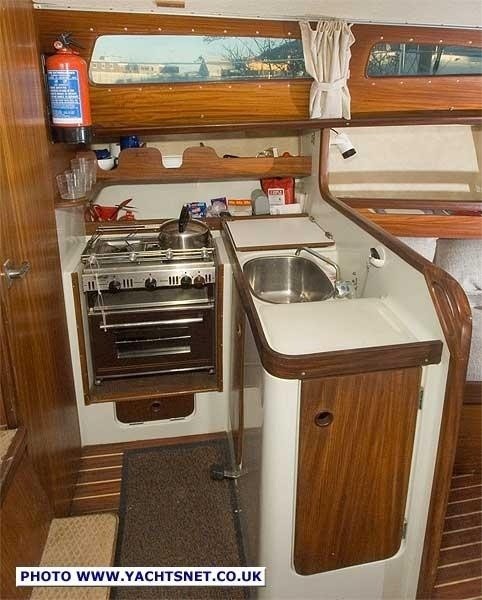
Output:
[294,246,342,299]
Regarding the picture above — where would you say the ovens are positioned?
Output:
[88,286,217,386]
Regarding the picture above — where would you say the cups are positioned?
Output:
[93,206,118,221]
[55,156,94,200]
[119,135,140,151]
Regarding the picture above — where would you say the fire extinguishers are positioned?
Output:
[40,29,94,144]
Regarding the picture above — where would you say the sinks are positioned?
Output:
[240,251,335,305]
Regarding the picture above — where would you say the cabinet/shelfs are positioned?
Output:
[0,0,84,518]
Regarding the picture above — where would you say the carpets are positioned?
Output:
[27,513,117,599]
[111,441,249,599]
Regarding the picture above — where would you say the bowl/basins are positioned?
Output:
[97,158,114,171]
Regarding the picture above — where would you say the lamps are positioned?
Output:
[330,128,357,161]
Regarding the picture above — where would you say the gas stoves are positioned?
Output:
[80,233,216,293]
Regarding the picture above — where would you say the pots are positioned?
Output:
[159,204,210,255]
